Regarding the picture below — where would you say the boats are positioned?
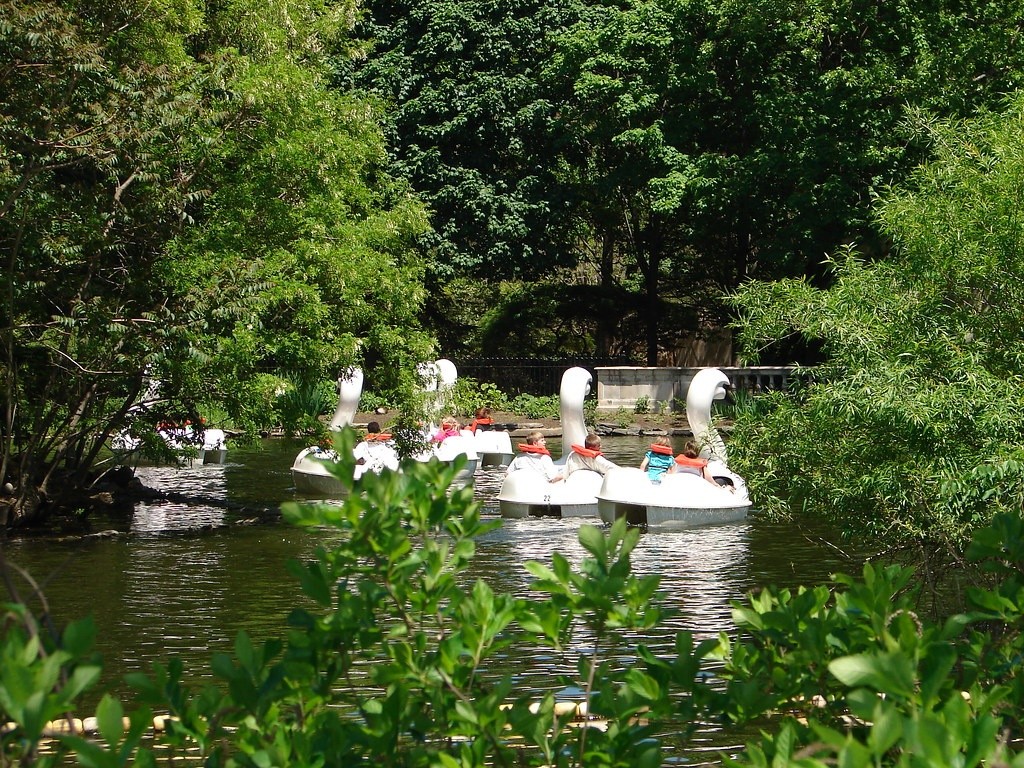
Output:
[404,364,481,475]
[435,358,516,466]
[496,366,596,519]
[111,376,228,465]
[594,367,753,534]
[289,364,405,496]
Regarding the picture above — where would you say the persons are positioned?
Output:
[639,436,676,474]
[363,422,392,443]
[431,416,462,449]
[563,435,622,481]
[672,441,721,487]
[504,431,563,483]
[463,408,493,434]
